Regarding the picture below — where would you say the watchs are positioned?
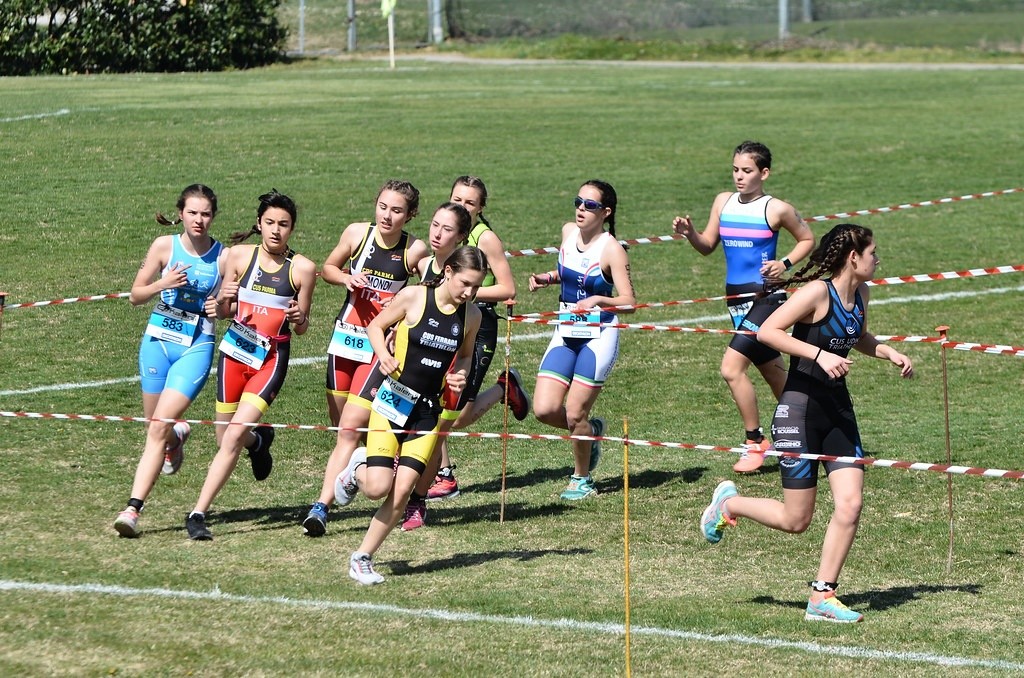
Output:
[779,256,794,271]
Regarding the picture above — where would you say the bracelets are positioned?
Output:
[298,316,306,325]
[814,346,824,363]
[541,271,557,289]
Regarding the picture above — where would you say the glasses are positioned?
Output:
[573,197,604,212]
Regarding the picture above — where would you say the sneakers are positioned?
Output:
[561,475,598,501]
[401,498,428,532]
[700,479,739,544]
[805,591,865,623]
[732,435,771,472]
[588,415,607,471]
[184,510,214,541]
[349,550,384,586]
[163,422,191,474]
[497,367,531,419]
[246,422,274,481]
[302,502,328,537]
[114,506,141,535]
[335,447,367,506]
[426,465,461,500]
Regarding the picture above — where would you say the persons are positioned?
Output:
[528,180,636,501]
[385,175,531,531]
[334,245,488,586]
[113,183,237,538]
[700,224,914,623]
[303,181,431,536]
[672,142,817,472]
[186,188,316,541]
